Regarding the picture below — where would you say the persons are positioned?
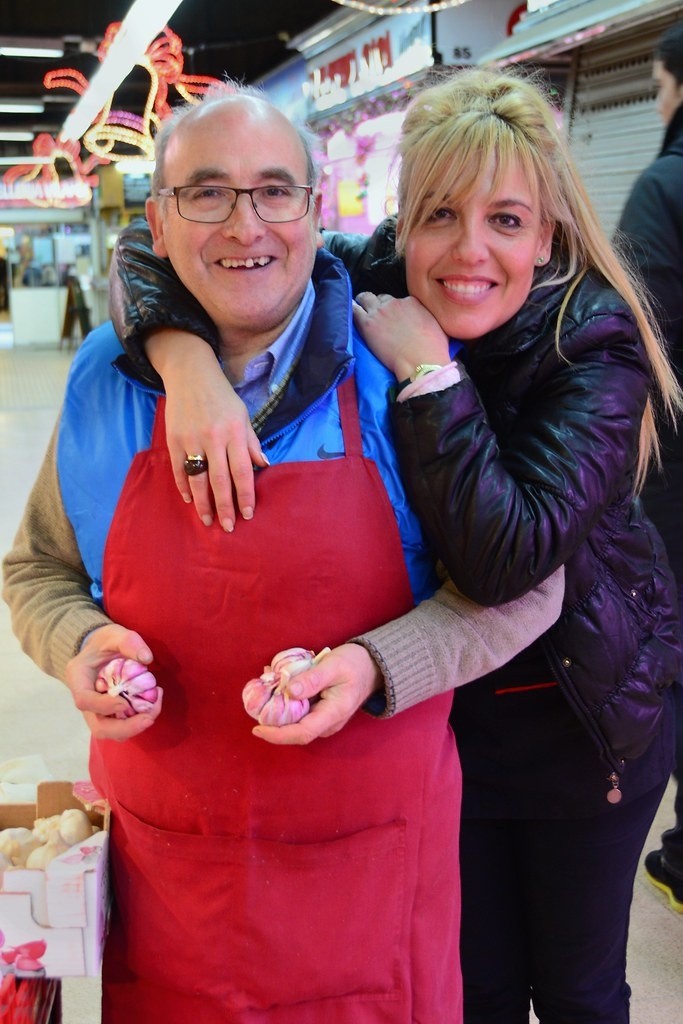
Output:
[0,85,566,1024]
[107,23,683,1024]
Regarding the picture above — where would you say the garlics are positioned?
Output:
[241,647,333,727]
[0,805,103,872]
[95,658,158,719]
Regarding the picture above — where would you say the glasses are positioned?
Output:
[157,184,313,224]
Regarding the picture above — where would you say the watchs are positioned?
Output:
[397,364,441,396]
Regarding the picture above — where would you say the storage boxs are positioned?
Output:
[0,781,111,977]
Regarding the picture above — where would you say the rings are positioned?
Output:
[182,455,209,475]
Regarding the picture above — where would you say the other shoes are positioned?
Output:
[645,850,683,916]
